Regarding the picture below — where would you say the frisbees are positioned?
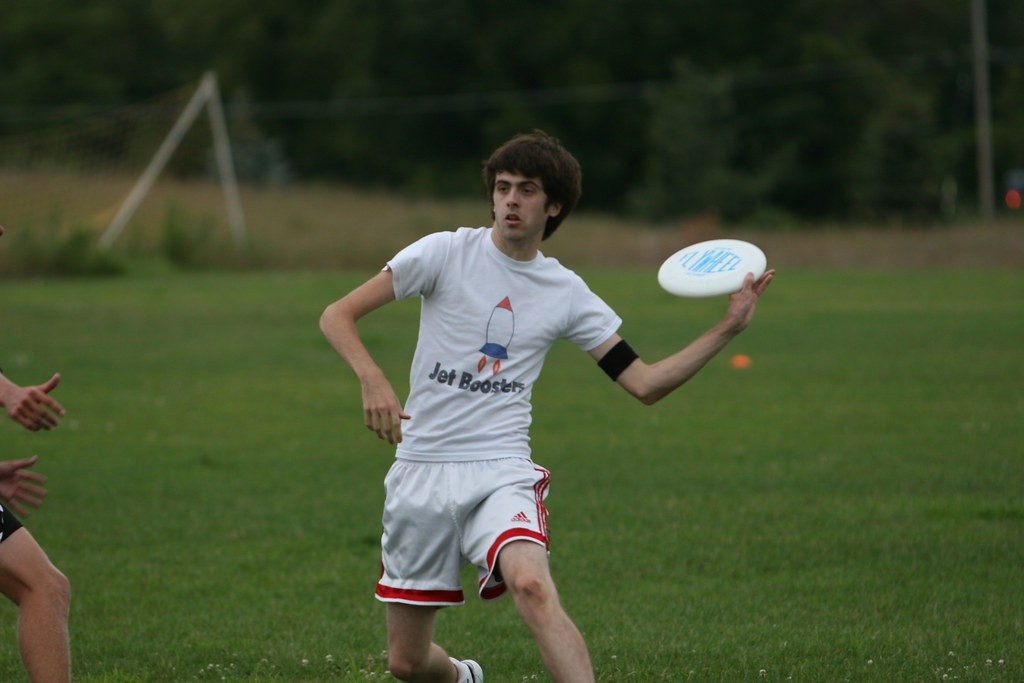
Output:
[658,239,767,298]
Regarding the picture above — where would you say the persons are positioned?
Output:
[0,371,71,683]
[319,134,774,683]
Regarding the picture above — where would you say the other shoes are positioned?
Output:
[448,656,484,683]
